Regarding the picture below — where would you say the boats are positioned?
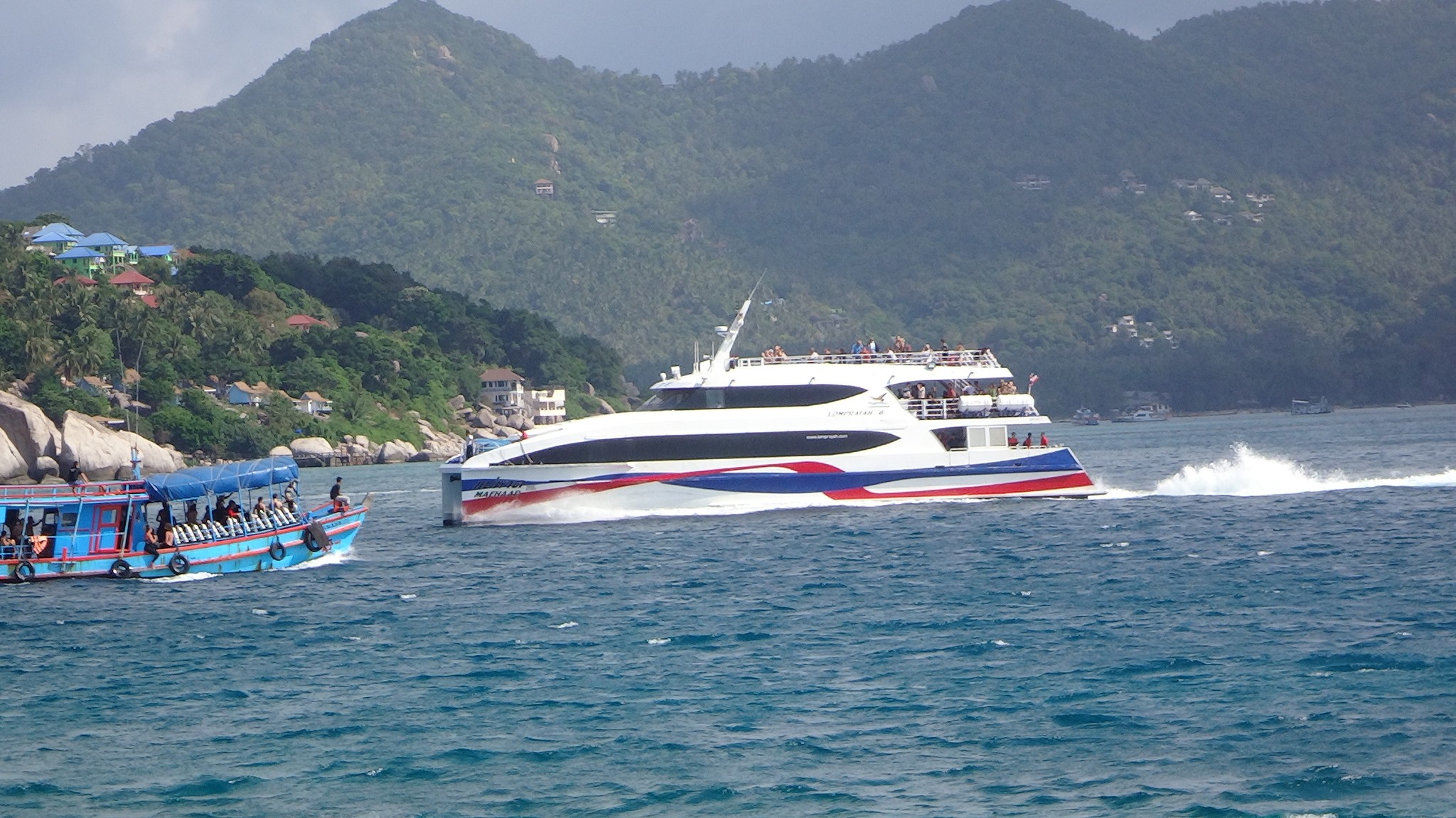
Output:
[1397,402,1412,408]
[442,267,1108,528]
[1071,405,1100,425]
[1290,395,1334,416]
[0,328,372,584]
[1109,403,1172,423]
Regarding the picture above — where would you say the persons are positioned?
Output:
[466,430,474,459]
[144,481,300,568]
[515,429,529,442]
[25,515,44,536]
[13,518,26,541]
[330,477,352,511]
[67,462,93,497]
[730,329,1050,450]
[0,531,16,560]
